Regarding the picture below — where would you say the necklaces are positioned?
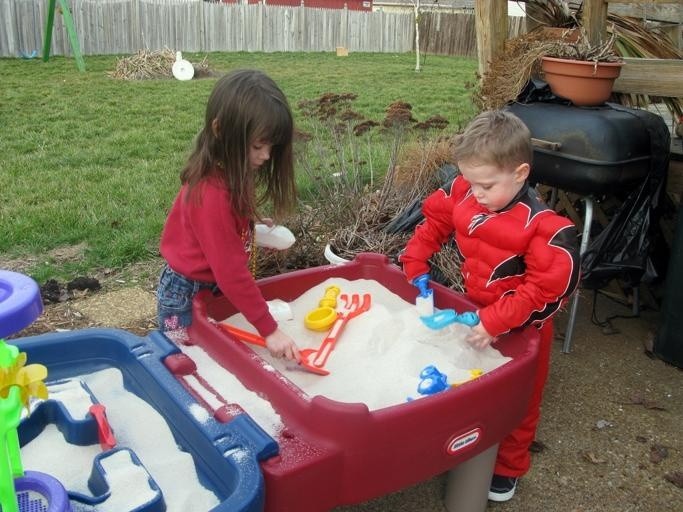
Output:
[210,161,256,280]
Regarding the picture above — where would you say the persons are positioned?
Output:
[153,69,307,366]
[398,108,583,503]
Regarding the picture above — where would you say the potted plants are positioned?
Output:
[471,28,628,112]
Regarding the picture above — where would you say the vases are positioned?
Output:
[321,218,412,269]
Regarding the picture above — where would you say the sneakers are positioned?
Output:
[487,474,518,502]
